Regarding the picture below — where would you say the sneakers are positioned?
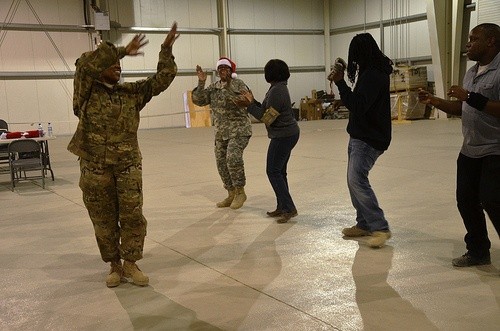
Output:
[342,226,368,237]
[368,230,391,247]
[453,253,491,267]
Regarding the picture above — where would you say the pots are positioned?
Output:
[21,130,38,138]
[4,132,24,139]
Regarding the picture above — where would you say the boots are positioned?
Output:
[106,260,122,286]
[216,189,235,208]
[122,260,149,285]
[230,188,247,209]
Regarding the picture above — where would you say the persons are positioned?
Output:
[331,33,393,247]
[67,22,180,286]
[234,59,300,224]
[417,23,500,269]
[192,57,251,209]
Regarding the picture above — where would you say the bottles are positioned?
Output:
[48,123,52,136]
[38,124,43,138]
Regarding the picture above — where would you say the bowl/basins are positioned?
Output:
[43,131,46,137]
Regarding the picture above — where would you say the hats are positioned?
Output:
[216,57,237,79]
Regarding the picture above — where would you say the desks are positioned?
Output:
[0,136,56,181]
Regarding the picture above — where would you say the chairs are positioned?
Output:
[8,139,45,192]
[0,119,8,136]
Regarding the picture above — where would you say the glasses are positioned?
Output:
[219,66,229,70]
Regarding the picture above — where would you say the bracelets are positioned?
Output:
[467,91,471,99]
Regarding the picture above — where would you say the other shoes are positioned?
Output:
[267,209,282,217]
[278,209,298,223]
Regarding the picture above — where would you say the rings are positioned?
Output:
[452,89,453,92]
